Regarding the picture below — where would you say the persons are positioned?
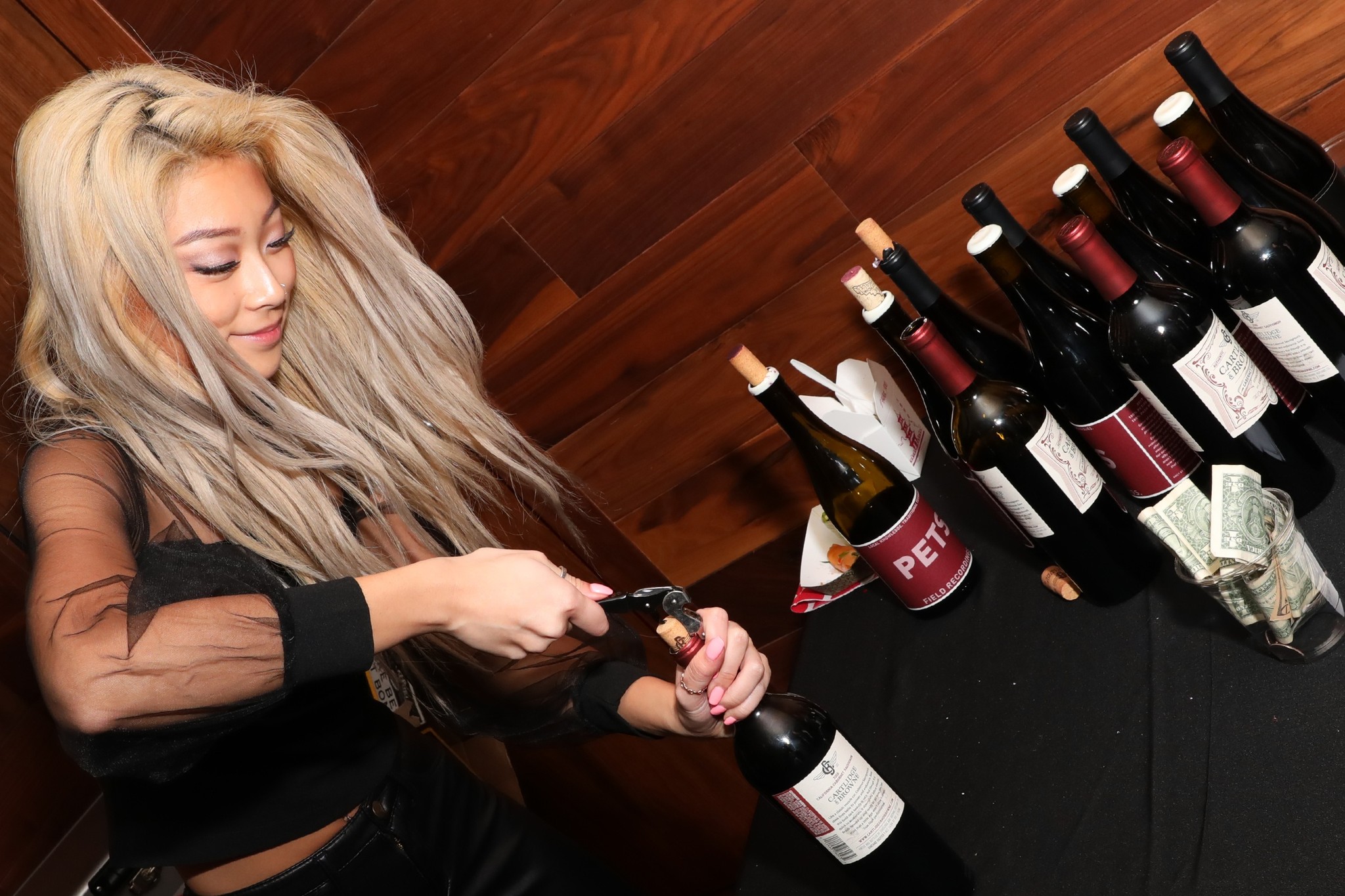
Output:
[14,63,772,896]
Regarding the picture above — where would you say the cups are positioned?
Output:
[1174,487,1345,664]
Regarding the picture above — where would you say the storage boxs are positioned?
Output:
[798,357,931,482]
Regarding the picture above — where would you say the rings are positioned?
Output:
[680,671,708,695]
[558,566,567,578]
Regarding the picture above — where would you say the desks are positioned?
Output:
[733,432,1345,896]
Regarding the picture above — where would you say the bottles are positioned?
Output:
[727,344,981,615]
[1157,137,1345,428]
[854,218,1042,402]
[1052,163,1317,427]
[840,266,976,481]
[966,224,1213,507]
[1055,215,1336,519]
[1153,91,1345,268]
[656,614,918,883]
[1063,107,1230,271]
[962,182,1113,323]
[900,317,1158,608]
[1164,31,1345,227]
